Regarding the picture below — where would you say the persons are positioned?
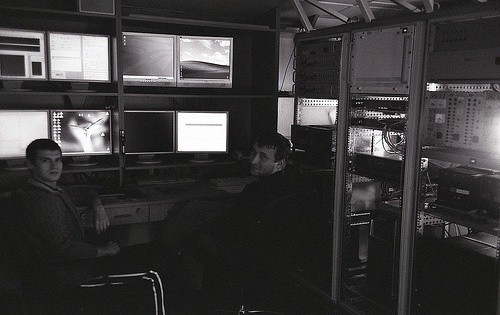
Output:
[0,139,173,315]
[185,131,327,302]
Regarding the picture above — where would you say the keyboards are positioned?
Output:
[211,176,259,186]
[135,176,195,185]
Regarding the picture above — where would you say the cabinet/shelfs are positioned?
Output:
[0,0,280,229]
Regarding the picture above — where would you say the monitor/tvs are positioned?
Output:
[78,0,116,15]
[0,27,233,170]
